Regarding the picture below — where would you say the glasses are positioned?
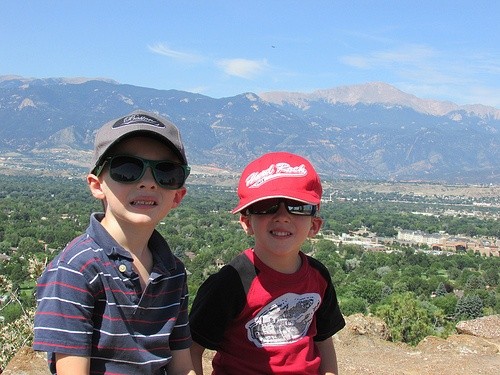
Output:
[240,198,316,216]
[94,154,190,190]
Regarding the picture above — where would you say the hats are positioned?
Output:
[230,152,322,215]
[89,110,187,174]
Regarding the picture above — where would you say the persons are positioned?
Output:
[33,108,196,375]
[189,152,347,375]
